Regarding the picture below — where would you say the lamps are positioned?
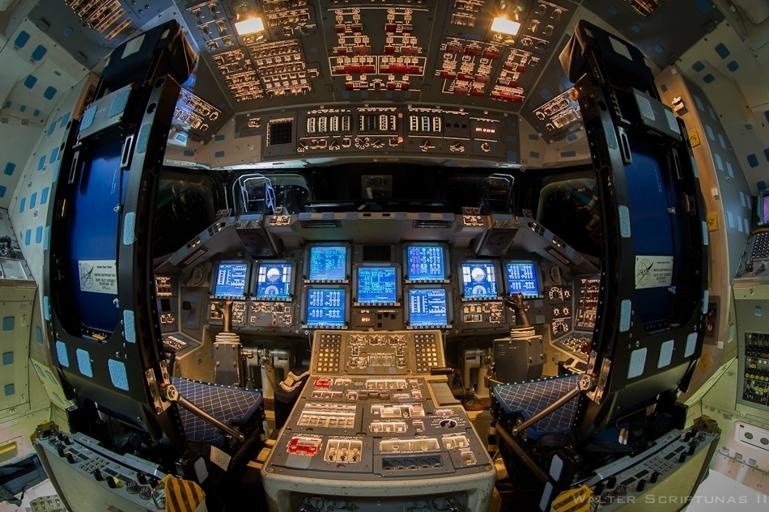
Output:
[148,257,264,471]
[490,263,612,489]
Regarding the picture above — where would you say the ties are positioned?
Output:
[403,284,455,329]
[402,240,452,283]
[302,240,352,284]
[351,262,403,307]
[209,259,251,301]
[502,259,545,299]
[455,258,504,298]
[299,284,351,330]
[248,259,297,302]
